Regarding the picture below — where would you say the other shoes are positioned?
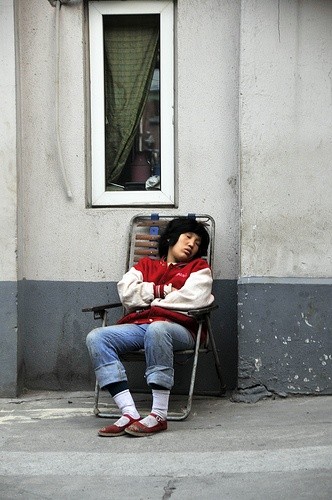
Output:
[97,413,167,436]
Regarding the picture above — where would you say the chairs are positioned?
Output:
[82,213,226,421]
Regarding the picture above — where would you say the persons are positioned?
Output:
[86,218,215,436]
